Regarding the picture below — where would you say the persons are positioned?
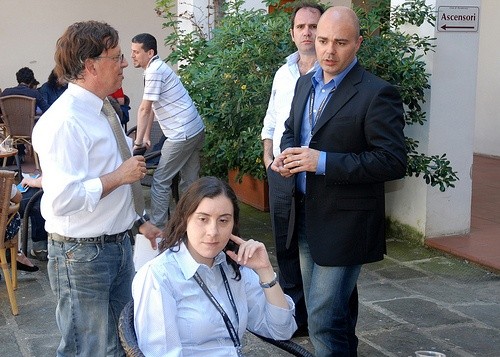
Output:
[261,4,326,340]
[129,31,205,232]
[279,7,406,356]
[31,21,165,357]
[109,86,124,106]
[131,177,298,357]
[4,171,40,271]
[20,174,44,242]
[0,67,67,162]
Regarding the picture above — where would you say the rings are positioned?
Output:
[297,162,301,165]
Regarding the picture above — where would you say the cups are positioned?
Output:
[5,138,13,152]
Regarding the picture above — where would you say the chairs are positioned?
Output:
[0,170,18,315]
[0,95,40,169]
[120,95,180,220]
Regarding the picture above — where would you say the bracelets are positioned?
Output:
[133,140,142,146]
[134,214,150,231]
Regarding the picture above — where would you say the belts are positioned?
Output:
[48,230,131,242]
[294,195,306,207]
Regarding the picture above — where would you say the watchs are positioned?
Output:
[259,273,278,289]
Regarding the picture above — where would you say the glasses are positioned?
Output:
[91,52,124,62]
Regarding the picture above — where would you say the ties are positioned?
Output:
[101,100,145,216]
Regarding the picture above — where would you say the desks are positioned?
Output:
[0,148,20,170]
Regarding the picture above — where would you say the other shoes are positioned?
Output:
[10,259,39,272]
[290,324,308,340]
[31,248,48,260]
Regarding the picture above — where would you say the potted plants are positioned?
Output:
[154,0,461,210]
